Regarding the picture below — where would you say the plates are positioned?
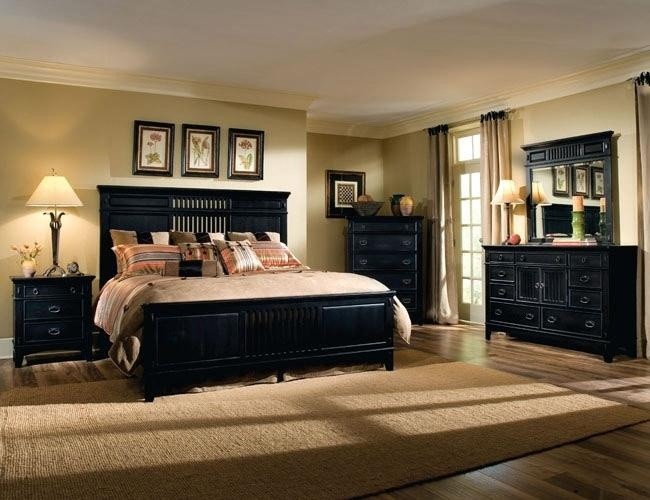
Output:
[530,238,555,243]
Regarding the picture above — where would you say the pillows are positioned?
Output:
[227,231,281,242]
[213,240,311,273]
[168,229,226,246]
[110,229,170,279]
[111,244,224,282]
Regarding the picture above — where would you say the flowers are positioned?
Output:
[238,140,252,170]
[145,133,163,164]
[10,242,44,264]
[192,135,210,166]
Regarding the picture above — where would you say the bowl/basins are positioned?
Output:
[349,202,384,217]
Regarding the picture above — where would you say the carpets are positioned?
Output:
[0,361,650,500]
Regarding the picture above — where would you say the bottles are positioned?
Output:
[391,194,405,217]
[401,195,414,216]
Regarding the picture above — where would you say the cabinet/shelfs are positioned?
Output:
[345,216,424,321]
[481,243,638,363]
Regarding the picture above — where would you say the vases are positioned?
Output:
[22,257,36,277]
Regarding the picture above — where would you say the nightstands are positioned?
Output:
[9,274,96,368]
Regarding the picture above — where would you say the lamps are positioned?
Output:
[25,175,84,276]
[532,181,552,238]
[489,180,526,244]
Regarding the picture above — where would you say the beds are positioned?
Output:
[93,185,412,402]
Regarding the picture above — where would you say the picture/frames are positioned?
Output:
[552,165,604,200]
[325,169,366,218]
[132,119,175,177]
[227,127,264,180]
[181,123,220,178]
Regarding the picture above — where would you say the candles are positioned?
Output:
[572,195,584,211]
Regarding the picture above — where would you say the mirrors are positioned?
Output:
[520,131,615,240]
